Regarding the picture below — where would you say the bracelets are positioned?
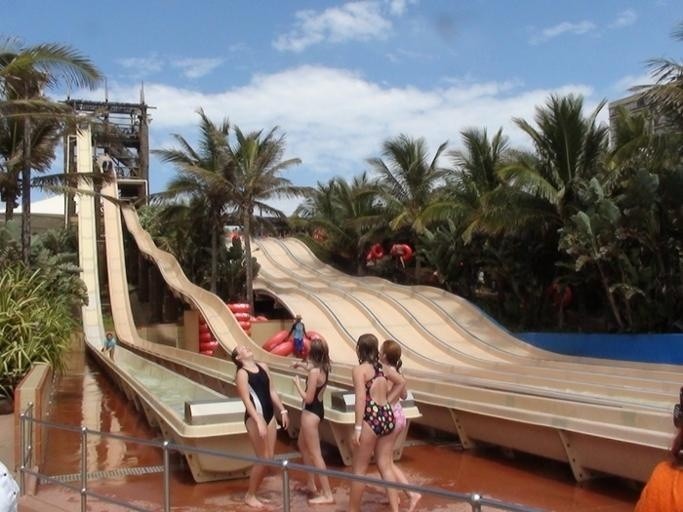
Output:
[281,410,288,414]
[354,426,362,430]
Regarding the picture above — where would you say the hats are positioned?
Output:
[294,315,302,319]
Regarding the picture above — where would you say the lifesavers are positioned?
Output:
[263,328,328,360]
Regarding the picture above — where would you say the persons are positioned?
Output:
[380,340,421,512]
[635,389,683,512]
[284,315,312,358]
[231,346,289,509]
[291,337,335,506]
[101,333,116,360]
[348,334,405,512]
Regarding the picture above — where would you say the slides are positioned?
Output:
[77,113,423,485]
[221,237,682,483]
